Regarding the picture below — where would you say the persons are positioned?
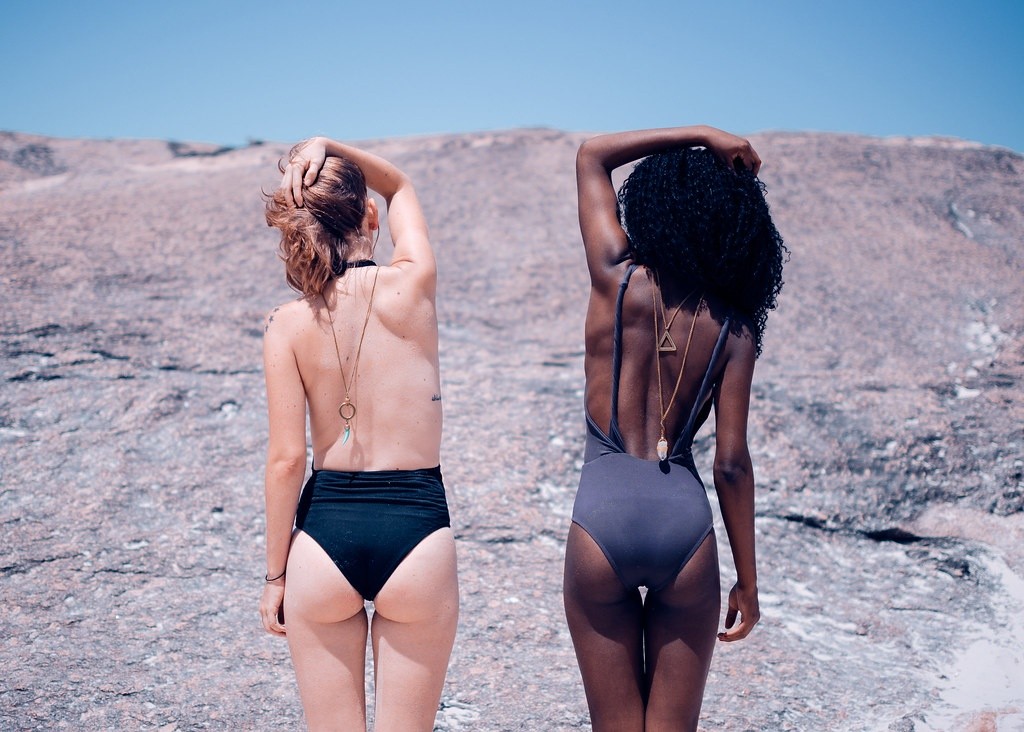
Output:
[262,137,459,732]
[565,126,787,732]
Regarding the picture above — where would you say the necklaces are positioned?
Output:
[349,260,376,266]
[320,266,381,446]
[652,274,703,461]
[657,272,691,352]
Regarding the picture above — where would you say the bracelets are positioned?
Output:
[266,571,287,581]
